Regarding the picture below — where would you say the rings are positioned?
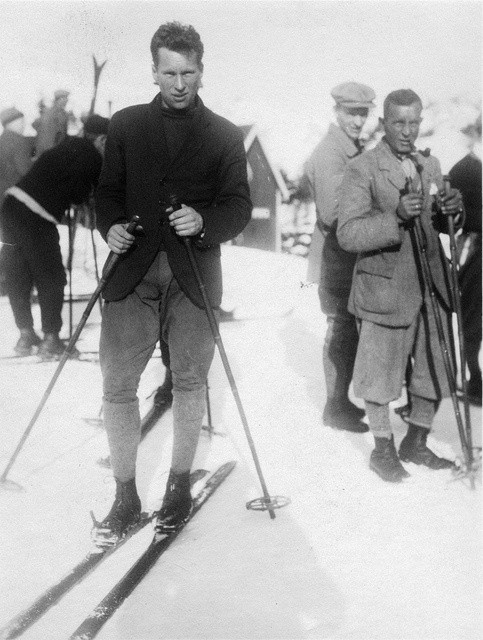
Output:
[415,204,419,209]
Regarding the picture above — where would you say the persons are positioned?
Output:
[31,90,70,159]
[337,89,465,483]
[0,107,35,299]
[0,115,111,359]
[93,22,252,543]
[440,114,482,399]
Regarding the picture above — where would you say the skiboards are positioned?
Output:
[0,460,239,639]
[0,348,103,365]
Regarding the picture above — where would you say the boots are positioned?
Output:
[14,328,42,354]
[467,372,482,398]
[37,332,80,361]
[153,469,193,533]
[94,477,141,547]
[369,434,411,483]
[323,397,369,433]
[155,367,173,410]
[398,423,455,470]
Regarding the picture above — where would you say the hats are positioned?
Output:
[0,107,23,126]
[330,83,377,108]
[54,91,70,99]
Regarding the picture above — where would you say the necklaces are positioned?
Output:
[305,83,376,433]
